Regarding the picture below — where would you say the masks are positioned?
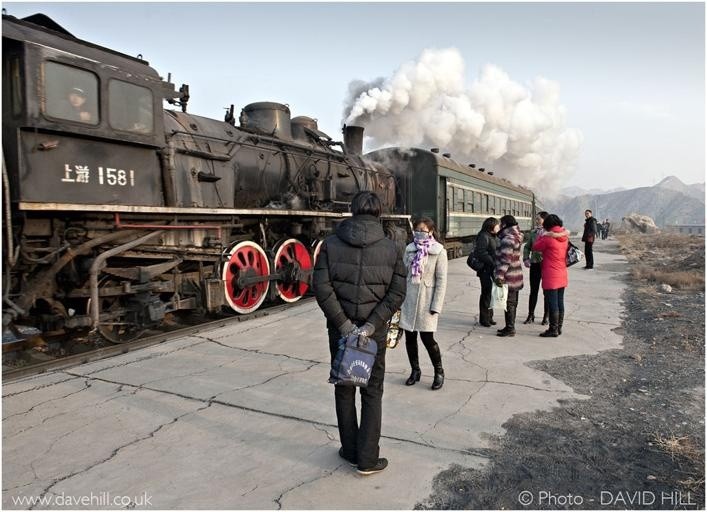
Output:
[411,230,433,244]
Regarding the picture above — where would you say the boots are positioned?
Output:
[404,342,421,388]
[425,343,446,392]
[540,291,564,338]
[495,306,518,340]
[522,288,538,325]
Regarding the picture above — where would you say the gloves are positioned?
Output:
[333,317,375,348]
[524,259,531,268]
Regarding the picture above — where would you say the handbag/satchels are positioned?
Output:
[466,253,486,273]
[488,281,509,313]
[566,239,583,270]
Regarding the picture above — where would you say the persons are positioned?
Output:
[581,208,611,271]
[396,216,447,390]
[472,210,570,338]
[48,83,95,125]
[311,192,406,476]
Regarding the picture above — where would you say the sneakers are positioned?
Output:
[479,319,495,330]
[337,444,391,478]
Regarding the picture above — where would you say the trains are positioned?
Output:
[2,8,548,347]
